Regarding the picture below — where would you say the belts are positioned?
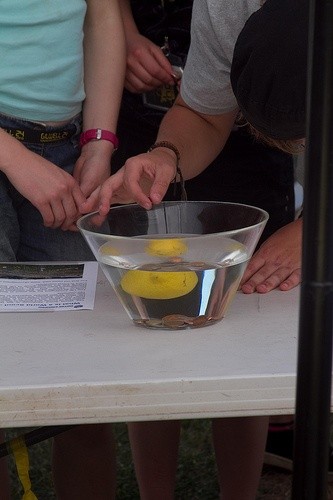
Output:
[2,125,81,145]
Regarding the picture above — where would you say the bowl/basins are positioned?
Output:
[76,200,270,329]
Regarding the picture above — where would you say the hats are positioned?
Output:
[233,5,308,139]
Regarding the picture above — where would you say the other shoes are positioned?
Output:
[264,429,333,477]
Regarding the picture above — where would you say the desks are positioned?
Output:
[0,261,300,500]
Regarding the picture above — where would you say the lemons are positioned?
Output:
[120,238,197,299]
